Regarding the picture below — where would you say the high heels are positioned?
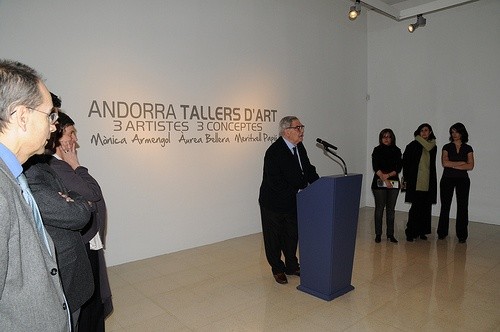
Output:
[375,234,381,242]
[387,235,398,242]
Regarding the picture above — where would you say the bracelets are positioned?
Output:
[453,162,454,167]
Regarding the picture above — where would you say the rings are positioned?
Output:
[65,150,68,153]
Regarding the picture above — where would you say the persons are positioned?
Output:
[49,111,114,332]
[437,123,474,243]
[23,92,95,332]
[258,116,319,284]
[0,59,73,332]
[371,128,403,243]
[400,123,437,241]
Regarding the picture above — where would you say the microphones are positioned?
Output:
[316,138,337,150]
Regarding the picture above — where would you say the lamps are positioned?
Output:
[409,15,426,33]
[348,3,361,20]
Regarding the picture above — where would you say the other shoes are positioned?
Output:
[406,234,413,242]
[420,234,428,240]
[438,234,445,240]
[459,237,465,243]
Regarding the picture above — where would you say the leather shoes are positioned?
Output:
[291,266,300,276]
[274,272,288,284]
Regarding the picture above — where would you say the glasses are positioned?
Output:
[286,125,304,131]
[10,107,59,124]
[382,135,391,138]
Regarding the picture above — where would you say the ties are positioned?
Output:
[293,147,301,170]
